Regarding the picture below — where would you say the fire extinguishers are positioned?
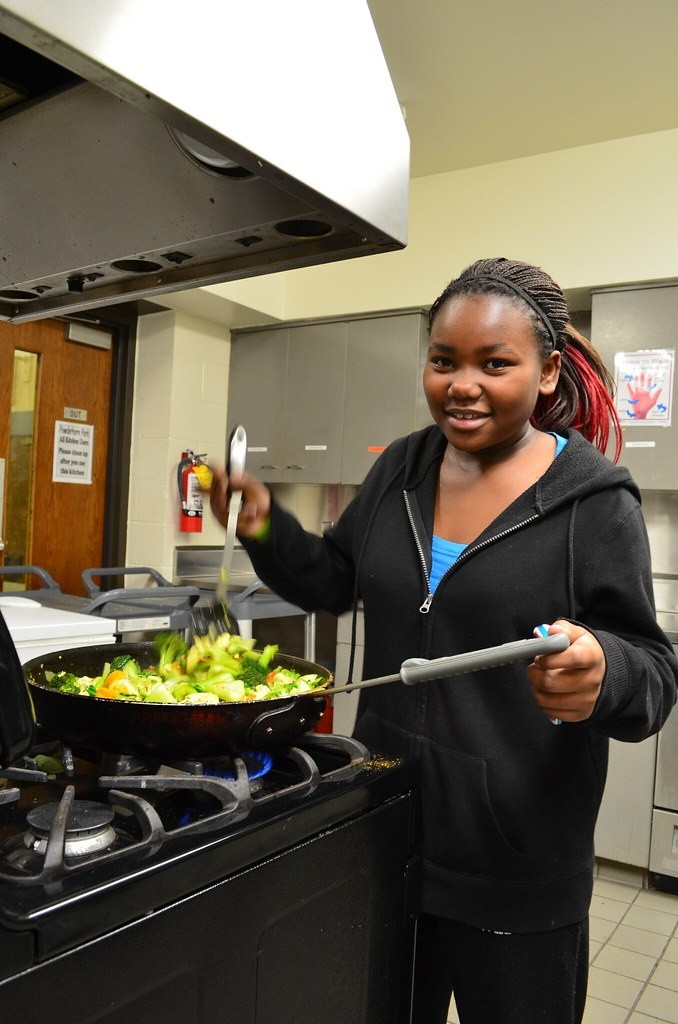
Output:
[174,448,210,534]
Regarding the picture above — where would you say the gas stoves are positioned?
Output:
[0,608,422,930]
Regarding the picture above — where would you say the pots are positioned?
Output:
[19,634,570,760]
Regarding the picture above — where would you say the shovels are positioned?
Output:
[189,423,249,640]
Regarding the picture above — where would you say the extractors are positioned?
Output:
[3,0,421,325]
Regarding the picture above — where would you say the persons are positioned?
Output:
[211,257,678,1024]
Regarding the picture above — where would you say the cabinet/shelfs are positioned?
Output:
[332,605,678,893]
[339,309,436,487]
[223,313,345,484]
[589,282,678,490]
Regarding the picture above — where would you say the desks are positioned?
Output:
[183,570,316,595]
